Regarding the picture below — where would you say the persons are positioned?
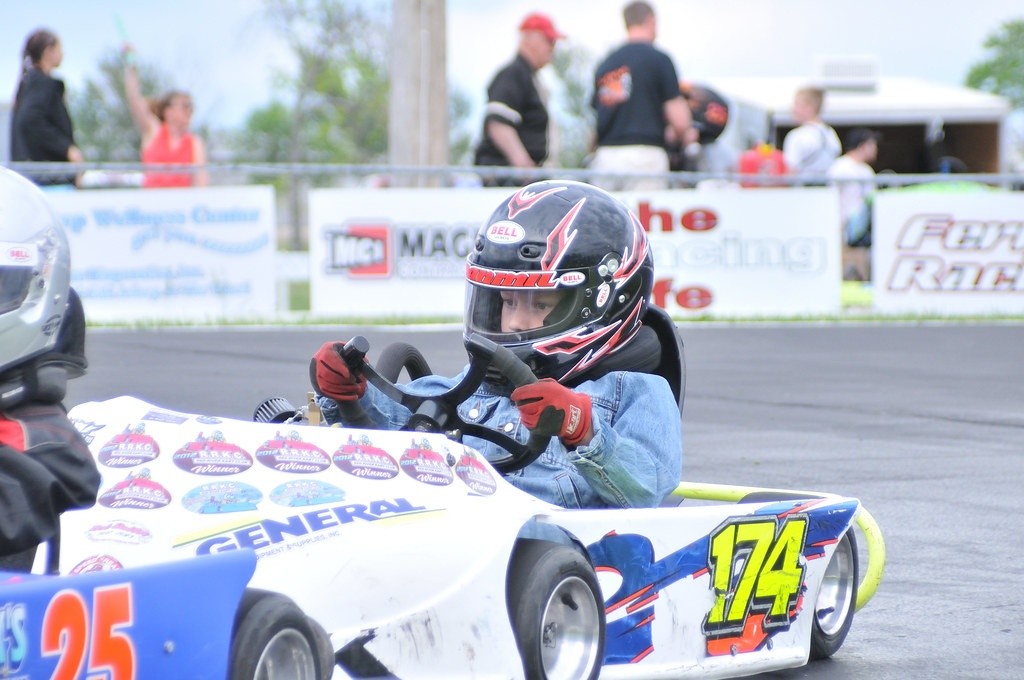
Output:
[474,15,564,186]
[0,165,102,554]
[826,127,883,247]
[125,66,209,187]
[310,179,683,508]
[588,0,695,190]
[9,31,83,184]
[784,88,842,175]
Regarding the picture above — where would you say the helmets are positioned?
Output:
[0,165,71,373]
[463,178,654,395]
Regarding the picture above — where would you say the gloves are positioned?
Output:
[510,377,592,445]
[309,342,369,402]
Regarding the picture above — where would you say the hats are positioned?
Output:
[520,12,568,41]
